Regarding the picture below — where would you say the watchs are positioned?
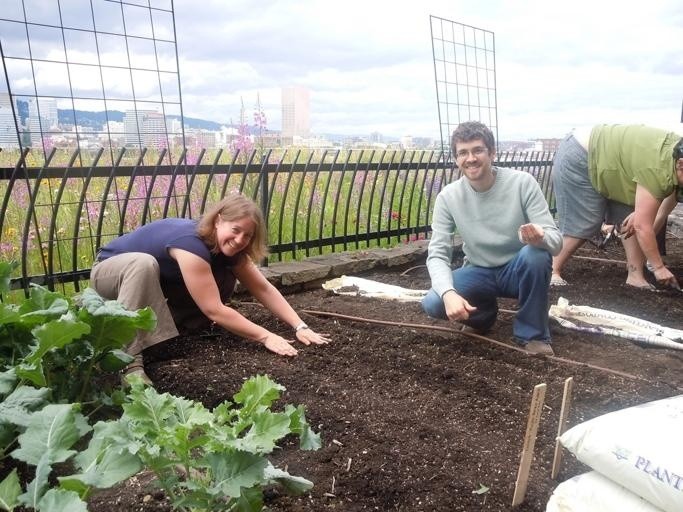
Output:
[296,321,308,331]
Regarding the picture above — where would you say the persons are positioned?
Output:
[88,193,332,387]
[550,122,683,292]
[645,217,667,273]
[421,121,564,357]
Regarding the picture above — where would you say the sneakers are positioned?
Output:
[524,339,556,359]
[117,362,155,397]
[460,324,484,341]
